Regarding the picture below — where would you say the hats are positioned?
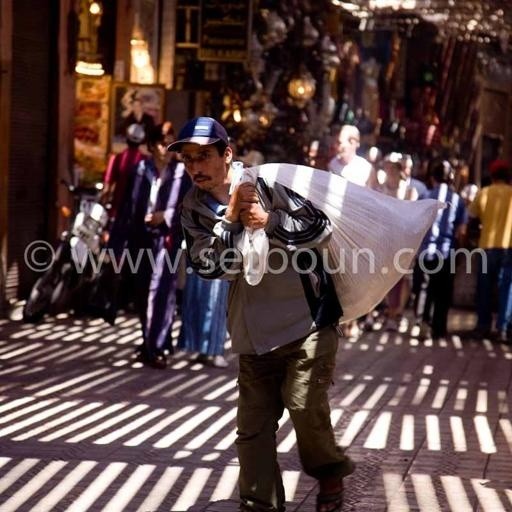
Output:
[383,153,406,172]
[486,159,509,176]
[167,117,230,151]
[239,151,264,166]
[127,125,145,143]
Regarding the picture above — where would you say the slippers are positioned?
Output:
[316,479,342,512]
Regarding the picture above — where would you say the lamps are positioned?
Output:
[285,62,317,109]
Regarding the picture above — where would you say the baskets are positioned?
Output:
[72,212,95,244]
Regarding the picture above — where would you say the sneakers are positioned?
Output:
[365,318,375,332]
[198,353,228,369]
[137,350,166,368]
[384,319,398,333]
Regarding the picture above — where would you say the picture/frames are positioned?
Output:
[108,82,167,146]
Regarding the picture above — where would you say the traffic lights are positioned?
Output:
[417,63,441,87]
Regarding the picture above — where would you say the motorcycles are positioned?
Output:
[25,178,116,322]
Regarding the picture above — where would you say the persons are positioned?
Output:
[166,116,357,509]
[90,0,512,368]
